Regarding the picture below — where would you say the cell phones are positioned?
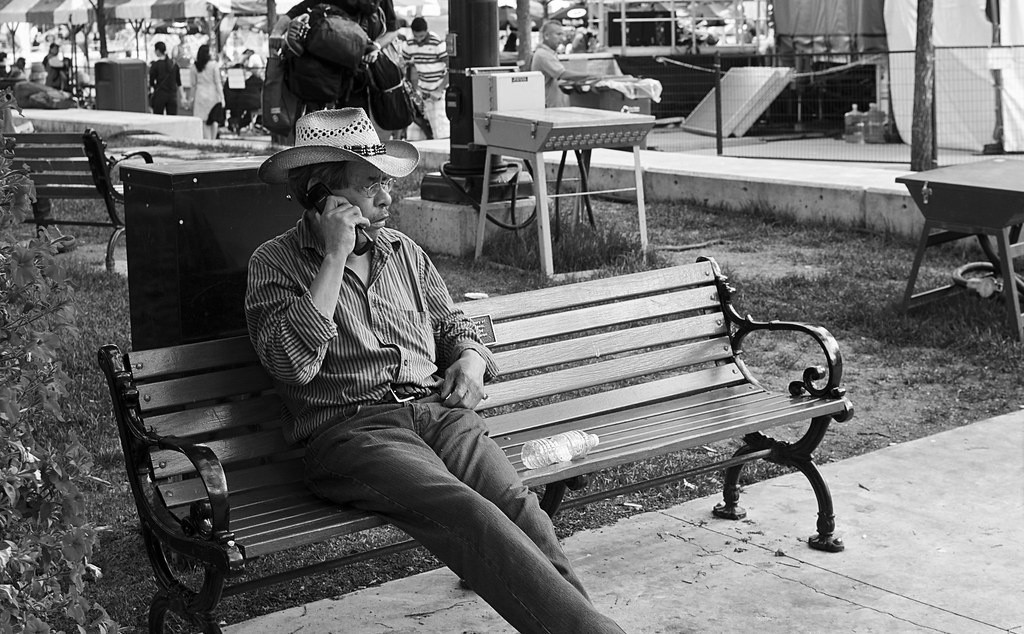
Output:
[307,183,374,256]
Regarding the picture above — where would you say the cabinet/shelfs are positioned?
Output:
[118,157,306,352]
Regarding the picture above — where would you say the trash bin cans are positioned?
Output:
[557,76,656,153]
[93,58,148,113]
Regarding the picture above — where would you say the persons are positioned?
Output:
[245,106,626,634]
[0,0,592,141]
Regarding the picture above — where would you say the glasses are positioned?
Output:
[358,178,392,198]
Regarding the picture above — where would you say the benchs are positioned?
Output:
[1,128,154,272]
[98,256,853,634]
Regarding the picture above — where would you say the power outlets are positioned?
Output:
[445,34,456,56]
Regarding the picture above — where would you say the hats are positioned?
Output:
[257,108,419,185]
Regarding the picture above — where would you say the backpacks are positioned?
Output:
[366,45,416,130]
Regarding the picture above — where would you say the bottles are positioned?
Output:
[520,430,599,470]
[844,103,864,144]
[864,103,886,144]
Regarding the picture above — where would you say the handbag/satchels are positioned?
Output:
[148,93,155,106]
[262,58,298,134]
[281,3,374,104]
